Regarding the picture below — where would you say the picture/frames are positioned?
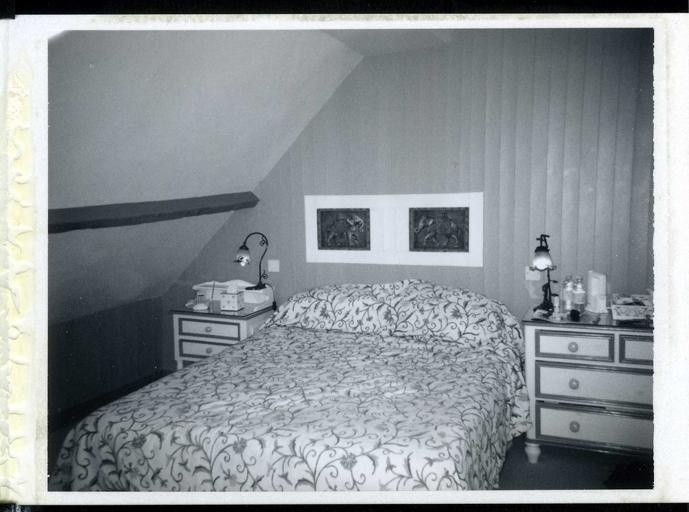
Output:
[304,193,483,266]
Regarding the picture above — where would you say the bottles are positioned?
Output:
[560,272,587,317]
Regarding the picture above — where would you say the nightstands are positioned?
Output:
[521,305,652,464]
[169,296,278,370]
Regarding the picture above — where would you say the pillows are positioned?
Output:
[274,281,502,344]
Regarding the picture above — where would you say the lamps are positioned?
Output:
[530,235,558,319]
[233,232,268,290]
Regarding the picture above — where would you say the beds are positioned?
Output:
[86,279,515,494]
